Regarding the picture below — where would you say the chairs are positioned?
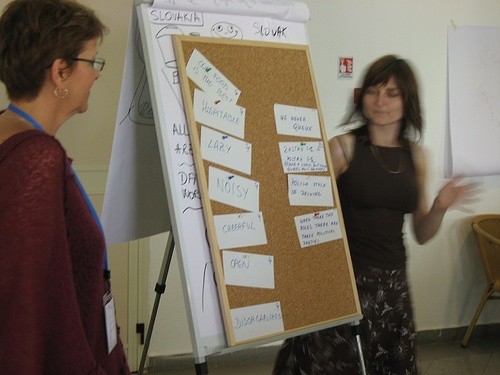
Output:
[461,214,500,349]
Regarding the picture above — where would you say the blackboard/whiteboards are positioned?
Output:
[129,0,365,361]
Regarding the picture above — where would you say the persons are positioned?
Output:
[273,55,482,375]
[1,0,131,375]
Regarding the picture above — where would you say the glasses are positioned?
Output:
[44,56,106,74]
